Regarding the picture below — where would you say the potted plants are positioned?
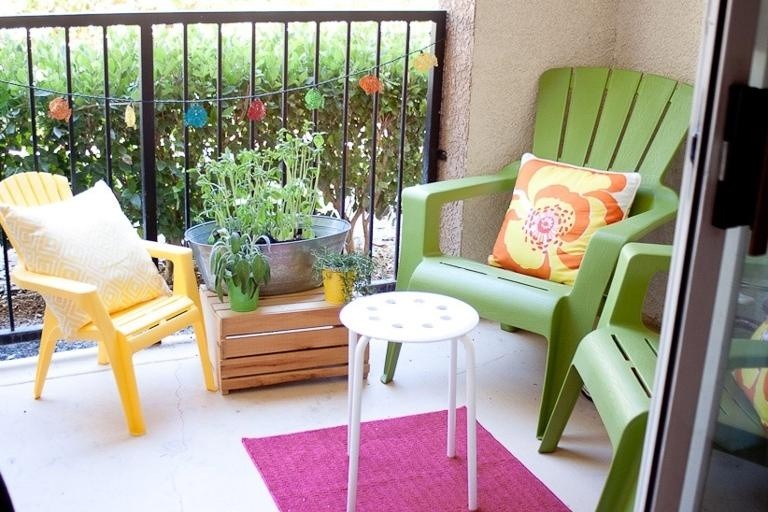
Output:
[180,131,379,313]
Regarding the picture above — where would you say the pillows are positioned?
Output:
[0,179,174,338]
[483,152,644,289]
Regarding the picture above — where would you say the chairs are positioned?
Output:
[0,171,218,437]
[535,239,768,511]
[381,69,698,437]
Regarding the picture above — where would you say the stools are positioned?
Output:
[341,291,483,512]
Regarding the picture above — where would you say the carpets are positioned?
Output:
[241,405,573,512]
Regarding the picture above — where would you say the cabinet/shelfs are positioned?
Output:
[200,284,371,395]
